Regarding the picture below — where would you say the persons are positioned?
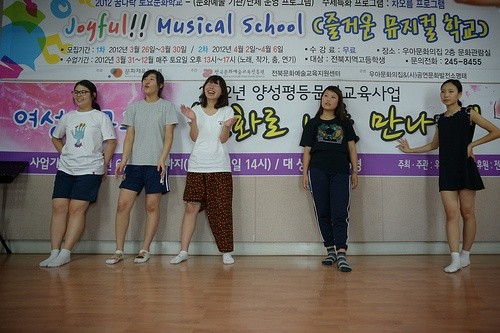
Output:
[169,75,238,263]
[39,79,115,267]
[300,86,359,272]
[396,79,500,273]
[106,70,179,264]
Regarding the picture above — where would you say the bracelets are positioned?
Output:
[351,171,358,174]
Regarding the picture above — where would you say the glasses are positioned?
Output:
[71,90,91,97]
